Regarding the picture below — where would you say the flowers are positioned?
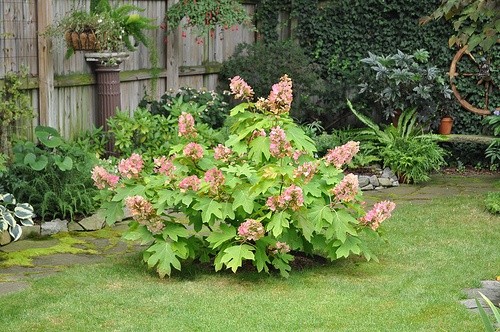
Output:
[58,12,398,271]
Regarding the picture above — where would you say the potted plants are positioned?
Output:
[358,50,452,128]
[346,99,449,184]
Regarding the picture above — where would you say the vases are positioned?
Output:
[437,116,453,135]
